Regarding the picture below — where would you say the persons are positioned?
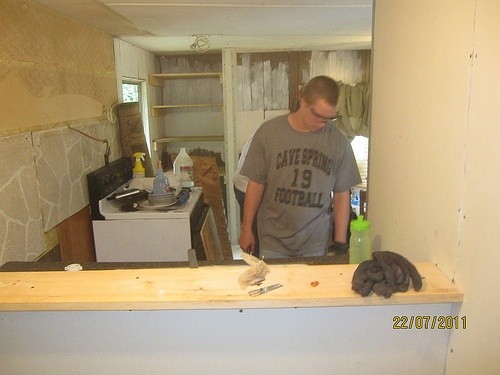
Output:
[238,75,362,257]
[232,130,259,258]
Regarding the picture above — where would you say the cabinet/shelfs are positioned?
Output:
[148,73,225,143]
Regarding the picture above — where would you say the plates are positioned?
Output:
[139,199,178,209]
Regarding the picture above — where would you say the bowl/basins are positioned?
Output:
[148,187,176,205]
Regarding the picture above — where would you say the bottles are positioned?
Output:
[153,164,169,194]
[349,214,372,263]
[173,148,194,188]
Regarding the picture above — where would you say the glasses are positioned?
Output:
[306,103,341,122]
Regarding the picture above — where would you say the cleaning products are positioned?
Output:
[132,151,146,178]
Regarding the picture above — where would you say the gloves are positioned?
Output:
[329,241,349,256]
